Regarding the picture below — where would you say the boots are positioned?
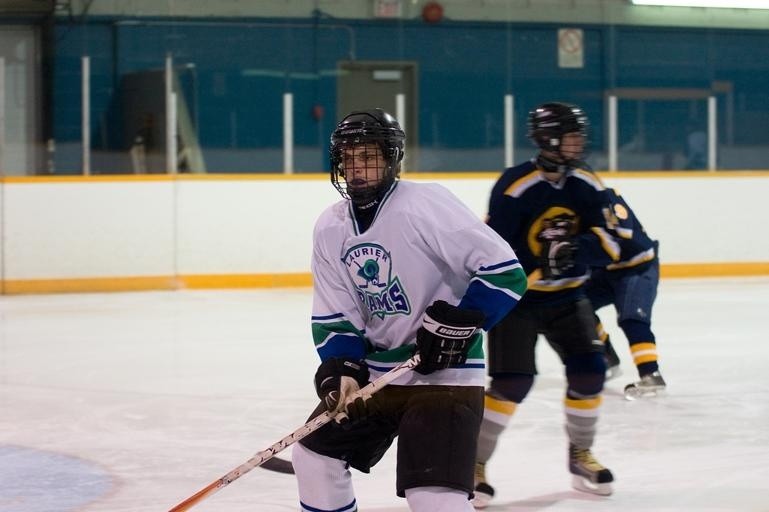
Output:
[601,334,620,370]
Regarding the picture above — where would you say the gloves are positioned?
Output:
[314,356,381,426]
[538,235,585,282]
[411,298,479,375]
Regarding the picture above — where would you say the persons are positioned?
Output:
[293,108,530,511]
[570,159,667,393]
[466,99,632,496]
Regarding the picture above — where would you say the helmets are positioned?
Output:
[529,101,588,153]
[328,106,407,205]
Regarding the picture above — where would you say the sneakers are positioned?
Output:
[623,370,667,394]
[470,462,495,498]
[567,442,614,484]
